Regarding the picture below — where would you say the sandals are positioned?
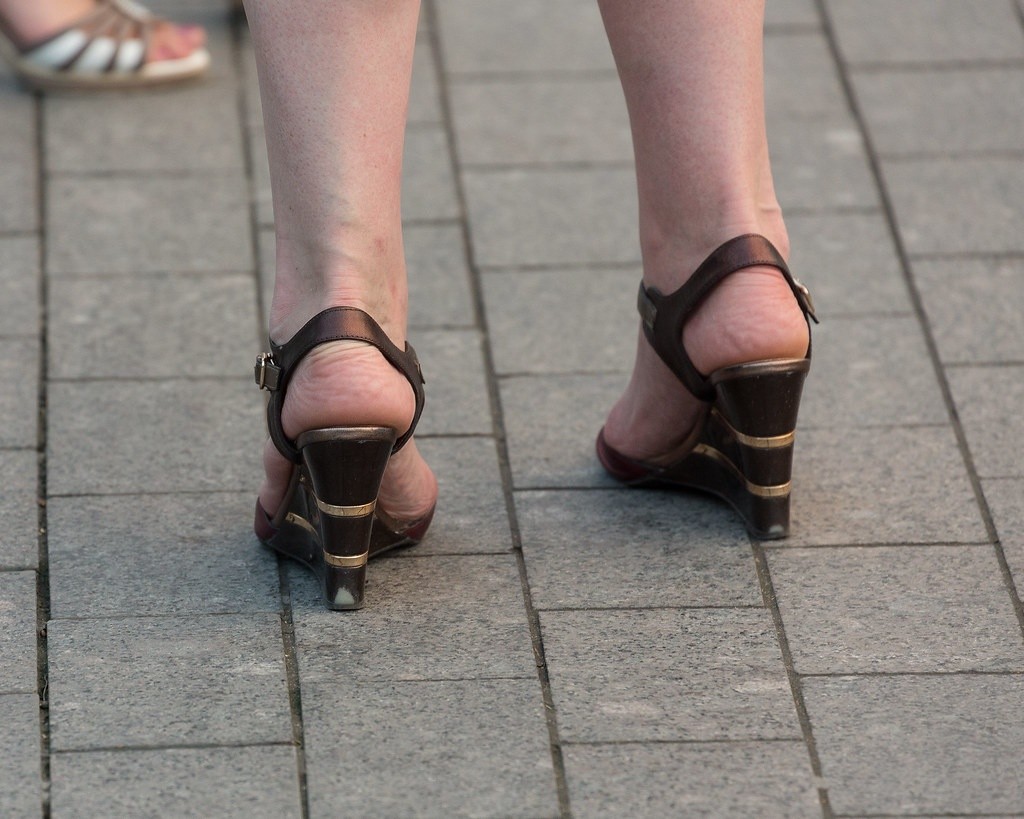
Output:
[1,0,211,93]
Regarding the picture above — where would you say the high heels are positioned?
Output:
[248,306,440,612]
[596,233,820,541]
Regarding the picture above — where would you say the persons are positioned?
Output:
[241,1,819,609]
[0,1,213,95]
